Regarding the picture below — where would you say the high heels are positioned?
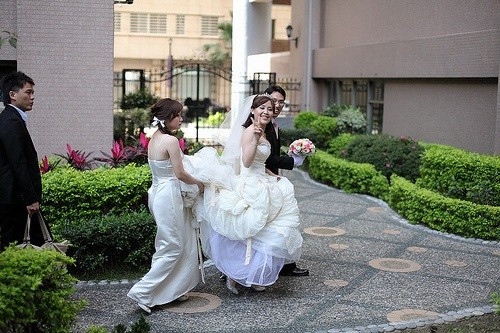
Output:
[138,303,151,313]
[253,287,266,292]
[226,277,238,294]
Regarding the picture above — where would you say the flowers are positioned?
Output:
[288,138,316,157]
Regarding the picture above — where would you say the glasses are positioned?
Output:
[274,98,287,105]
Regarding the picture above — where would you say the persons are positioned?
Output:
[220,84,309,281]
[126,97,205,315]
[0,71,54,255]
[181,92,303,295]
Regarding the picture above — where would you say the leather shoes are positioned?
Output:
[292,268,309,277]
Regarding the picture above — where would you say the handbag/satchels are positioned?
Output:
[14,211,70,271]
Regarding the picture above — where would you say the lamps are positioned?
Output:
[286,25,297,48]
